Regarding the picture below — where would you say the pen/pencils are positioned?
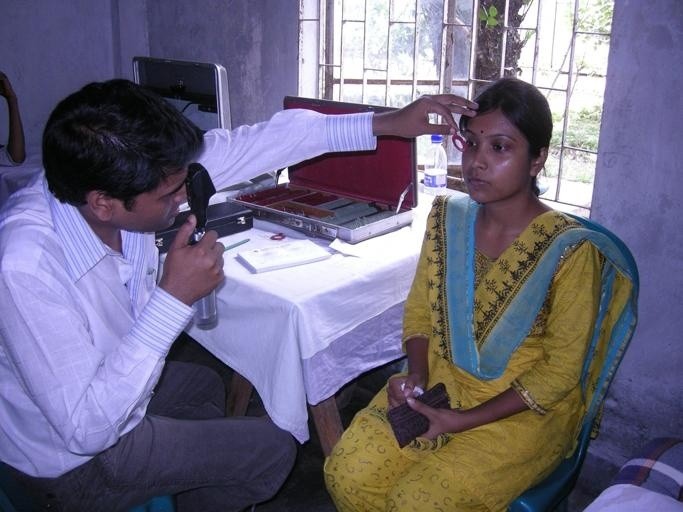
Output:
[224,238,250,251]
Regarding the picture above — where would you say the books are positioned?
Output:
[234,239,333,274]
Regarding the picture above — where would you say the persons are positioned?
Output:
[319,75,607,512]
[0,77,482,510]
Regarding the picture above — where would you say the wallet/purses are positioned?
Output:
[387,383,456,449]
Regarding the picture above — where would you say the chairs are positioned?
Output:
[0,490,178,512]
[509,212,640,511]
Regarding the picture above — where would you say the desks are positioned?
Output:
[2,147,471,460]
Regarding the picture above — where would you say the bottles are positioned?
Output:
[423,133,447,196]
